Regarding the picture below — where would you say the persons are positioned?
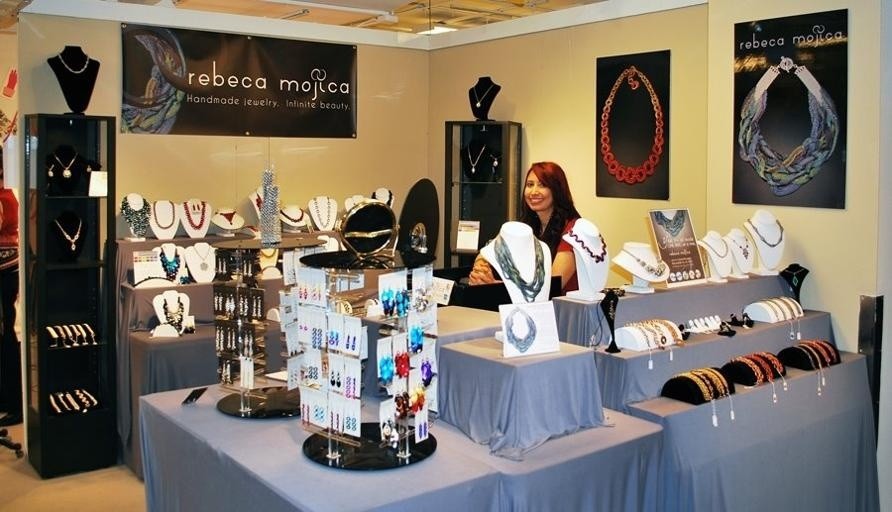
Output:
[1,146,23,431]
[468,160,585,303]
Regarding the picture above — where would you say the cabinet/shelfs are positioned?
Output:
[444,120,521,271]
[26,114,116,479]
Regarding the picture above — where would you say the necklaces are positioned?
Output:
[491,220,671,333]
[702,217,816,292]
[489,153,502,167]
[120,191,243,335]
[50,154,80,179]
[464,140,488,173]
[56,51,92,75]
[55,219,83,252]
[251,185,403,315]
[466,76,497,111]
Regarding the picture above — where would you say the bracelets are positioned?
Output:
[502,306,536,351]
[599,65,667,184]
[732,55,845,197]
[621,295,836,400]
[47,388,98,416]
[46,322,96,346]
